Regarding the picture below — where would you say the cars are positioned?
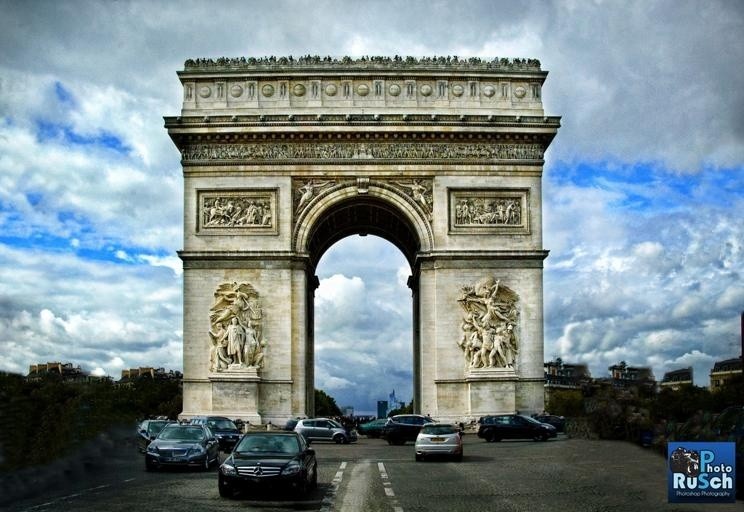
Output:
[358,412,468,461]
[135,414,245,473]
[293,418,358,446]
[218,430,319,501]
[476,410,570,443]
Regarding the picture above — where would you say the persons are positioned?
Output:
[458,279,519,369]
[294,178,330,215]
[456,201,521,225]
[328,414,369,428]
[395,179,432,217]
[209,292,263,370]
[204,198,271,226]
